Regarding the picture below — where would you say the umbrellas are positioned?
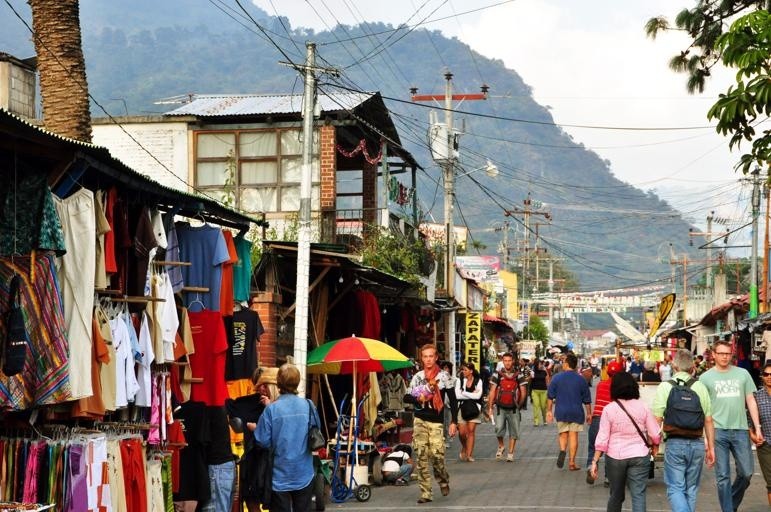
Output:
[306,335,416,466]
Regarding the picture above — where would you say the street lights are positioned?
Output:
[448,158,499,371]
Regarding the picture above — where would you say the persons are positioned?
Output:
[254,365,321,511]
[236,366,272,512]
[404,340,770,511]
[202,405,235,512]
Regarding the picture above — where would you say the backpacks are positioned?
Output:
[662,385,705,438]
[494,377,521,409]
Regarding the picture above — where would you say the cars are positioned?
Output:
[578,359,593,388]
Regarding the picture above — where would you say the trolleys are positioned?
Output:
[327,392,372,502]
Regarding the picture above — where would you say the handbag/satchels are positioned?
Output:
[307,426,326,451]
[648,461,655,479]
[460,399,480,421]
[2,307,28,377]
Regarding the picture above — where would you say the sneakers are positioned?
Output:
[586,465,595,484]
[507,451,514,462]
[496,445,505,458]
[396,478,409,486]
[603,477,611,487]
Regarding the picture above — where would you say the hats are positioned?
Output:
[644,360,655,372]
[255,367,280,386]
[498,352,505,357]
[607,362,623,376]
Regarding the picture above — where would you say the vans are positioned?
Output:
[601,355,616,381]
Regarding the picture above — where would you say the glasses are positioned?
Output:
[464,364,474,368]
[763,373,771,377]
[717,352,732,355]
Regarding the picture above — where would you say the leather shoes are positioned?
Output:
[467,457,475,462]
[459,453,465,461]
[418,499,432,503]
[441,484,449,496]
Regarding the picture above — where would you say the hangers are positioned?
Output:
[184,286,206,312]
[86,422,142,439]
[151,362,172,378]
[145,442,175,461]
[185,206,206,228]
[1,420,86,447]
[152,259,169,285]
[233,300,246,314]
[95,293,139,324]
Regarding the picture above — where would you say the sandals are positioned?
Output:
[557,450,566,468]
[569,464,581,470]
